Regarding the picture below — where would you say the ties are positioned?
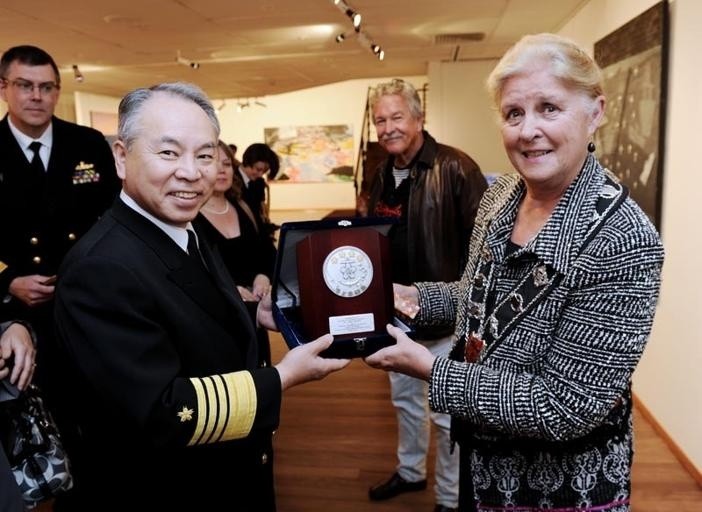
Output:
[186,230,205,272]
[25,143,46,175]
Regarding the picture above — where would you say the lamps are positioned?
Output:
[72,63,84,83]
[173,49,199,68]
[327,0,390,62]
[211,93,269,114]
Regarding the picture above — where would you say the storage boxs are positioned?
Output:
[271,213,423,369]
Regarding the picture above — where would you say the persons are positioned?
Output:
[1,45,119,309]
[228,144,243,168]
[0,321,40,512]
[234,142,281,218]
[352,77,491,512]
[198,139,273,368]
[362,32,668,512]
[49,82,352,511]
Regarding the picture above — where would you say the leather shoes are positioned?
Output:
[364,471,428,502]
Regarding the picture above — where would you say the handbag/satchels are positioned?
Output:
[0,385,73,497]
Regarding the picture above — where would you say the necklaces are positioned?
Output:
[202,198,231,216]
